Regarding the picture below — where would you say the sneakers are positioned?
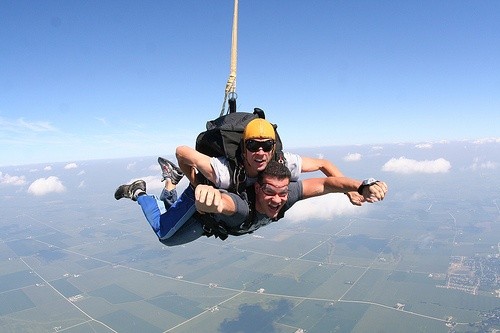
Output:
[115,180,146,201]
[158,157,183,185]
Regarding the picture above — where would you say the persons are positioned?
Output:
[114,157,385,247]
[174,119,368,207]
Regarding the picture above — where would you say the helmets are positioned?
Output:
[243,119,276,141]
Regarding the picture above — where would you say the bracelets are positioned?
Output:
[357,178,377,195]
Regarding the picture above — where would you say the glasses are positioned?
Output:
[260,184,289,196]
[247,140,276,152]
